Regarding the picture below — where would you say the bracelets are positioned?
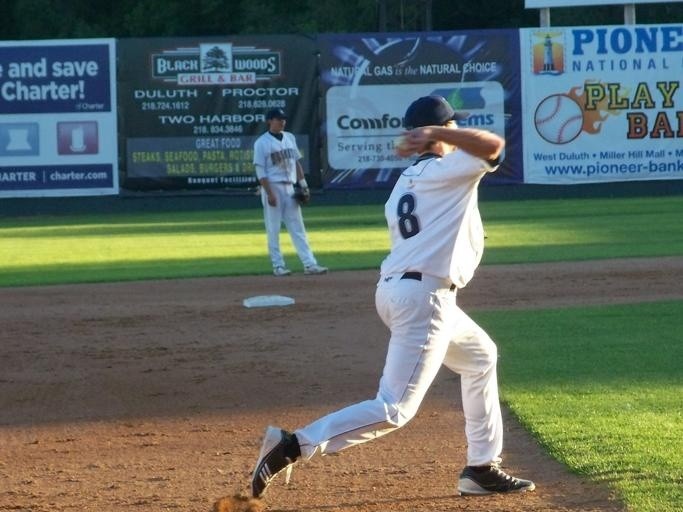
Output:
[299,179,308,189]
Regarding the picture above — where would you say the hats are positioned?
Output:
[404,96,468,129]
[265,109,288,120]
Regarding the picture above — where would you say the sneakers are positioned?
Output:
[305,265,328,275]
[458,465,536,496]
[251,425,297,497]
[272,268,290,277]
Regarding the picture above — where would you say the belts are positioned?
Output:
[402,272,422,281]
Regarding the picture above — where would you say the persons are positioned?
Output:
[252,107,330,277]
[247,94,538,500]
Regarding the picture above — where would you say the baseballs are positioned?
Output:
[392,135,410,153]
[535,95,584,144]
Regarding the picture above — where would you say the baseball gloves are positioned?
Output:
[291,186,311,205]
